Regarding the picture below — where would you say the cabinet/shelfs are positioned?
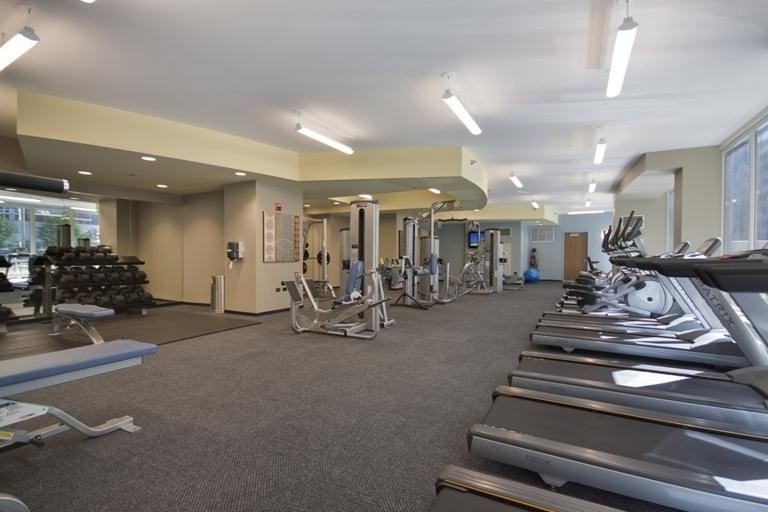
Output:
[23,246,156,322]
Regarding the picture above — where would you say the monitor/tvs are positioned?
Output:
[468,232,485,248]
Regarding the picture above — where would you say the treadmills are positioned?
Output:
[433,236,768,511]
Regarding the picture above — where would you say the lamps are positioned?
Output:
[585,0,640,208]
[0,9,39,71]
[442,88,540,211]
[294,110,354,156]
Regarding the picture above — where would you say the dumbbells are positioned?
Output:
[27,246,153,308]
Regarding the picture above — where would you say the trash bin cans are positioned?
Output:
[211,273,225,314]
